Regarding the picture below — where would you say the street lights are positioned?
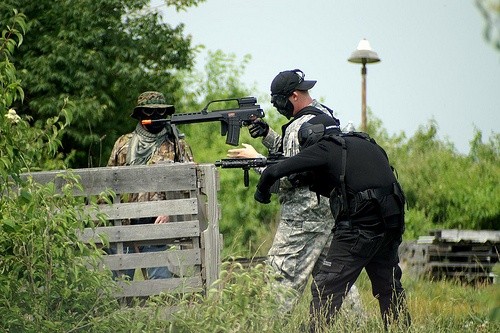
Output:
[347,37,381,133]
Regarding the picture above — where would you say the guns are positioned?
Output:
[215,148,291,194]
[141,97,265,146]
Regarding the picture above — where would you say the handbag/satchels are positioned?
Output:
[329,186,348,224]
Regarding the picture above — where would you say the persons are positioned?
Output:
[227,69,366,327]
[97,91,194,281]
[254,114,411,333]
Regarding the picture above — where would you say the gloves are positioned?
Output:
[288,171,309,187]
[247,117,269,139]
[254,190,272,204]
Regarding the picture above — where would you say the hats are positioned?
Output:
[306,114,340,135]
[131,91,176,119]
[271,71,317,91]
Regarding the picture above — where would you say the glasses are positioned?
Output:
[142,107,166,116]
